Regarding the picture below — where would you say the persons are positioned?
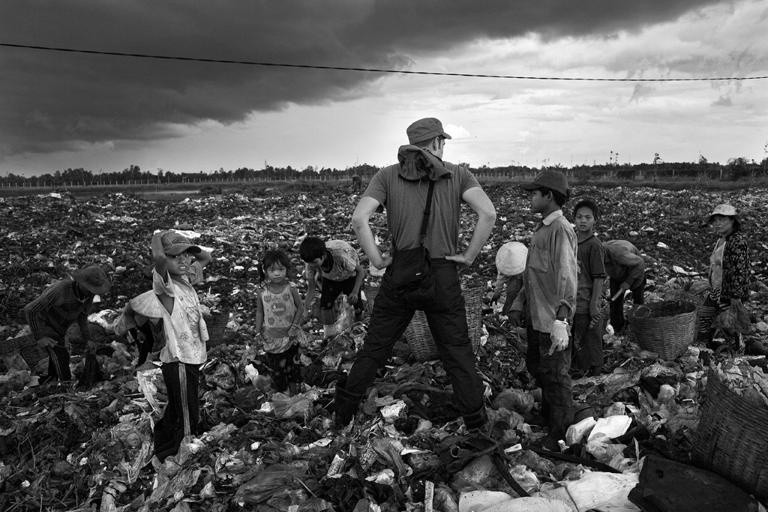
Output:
[23,266,111,382]
[599,240,647,333]
[520,170,578,449]
[254,245,306,397]
[350,174,362,190]
[491,239,529,327]
[302,238,366,340]
[703,203,751,348]
[335,116,496,439]
[147,229,213,462]
[571,201,609,377]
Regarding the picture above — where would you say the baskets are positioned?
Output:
[630,299,698,360]
[692,367,768,490]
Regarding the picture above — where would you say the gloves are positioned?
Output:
[547,319,570,354]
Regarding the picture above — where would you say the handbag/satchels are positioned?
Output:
[381,246,436,304]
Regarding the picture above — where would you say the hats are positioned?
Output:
[70,265,112,294]
[493,242,528,277]
[703,204,742,226]
[405,115,451,145]
[159,230,201,257]
[518,172,571,199]
